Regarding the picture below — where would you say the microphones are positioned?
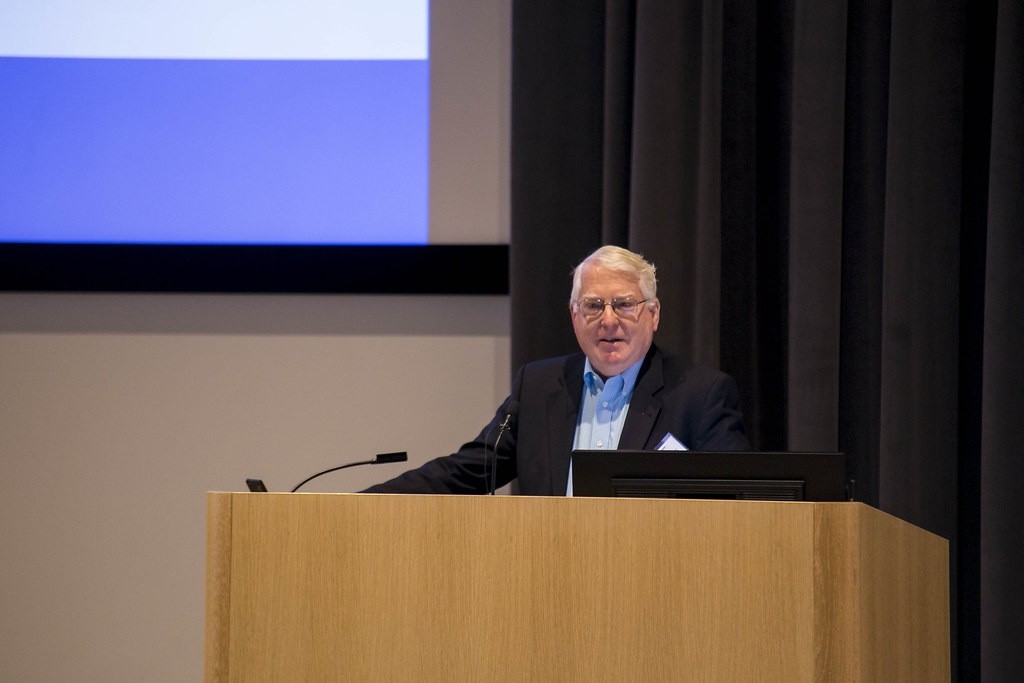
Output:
[490,401,520,495]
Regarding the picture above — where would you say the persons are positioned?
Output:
[352,245,765,499]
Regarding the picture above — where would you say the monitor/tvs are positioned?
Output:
[571,449,851,503]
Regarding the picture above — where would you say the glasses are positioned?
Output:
[578,297,647,315]
